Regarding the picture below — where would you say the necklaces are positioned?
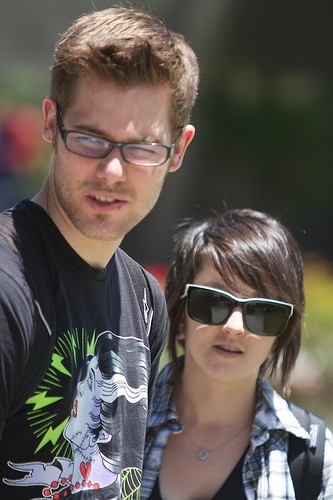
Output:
[176,417,256,462]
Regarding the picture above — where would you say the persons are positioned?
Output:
[0,0,202,500]
[137,196,333,500]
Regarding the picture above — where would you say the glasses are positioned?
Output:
[181,284,294,337]
[54,101,184,167]
